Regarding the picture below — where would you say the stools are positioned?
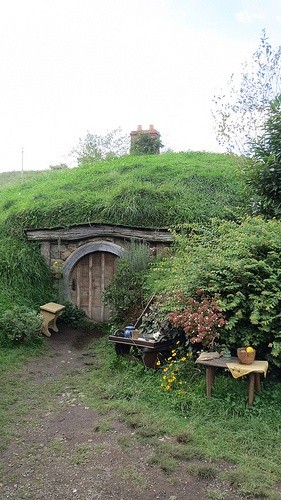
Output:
[37,302,66,337]
[195,351,269,408]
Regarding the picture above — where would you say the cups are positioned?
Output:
[130,330,139,339]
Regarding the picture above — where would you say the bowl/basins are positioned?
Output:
[237,348,256,365]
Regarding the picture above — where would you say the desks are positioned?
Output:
[105,328,172,369]
